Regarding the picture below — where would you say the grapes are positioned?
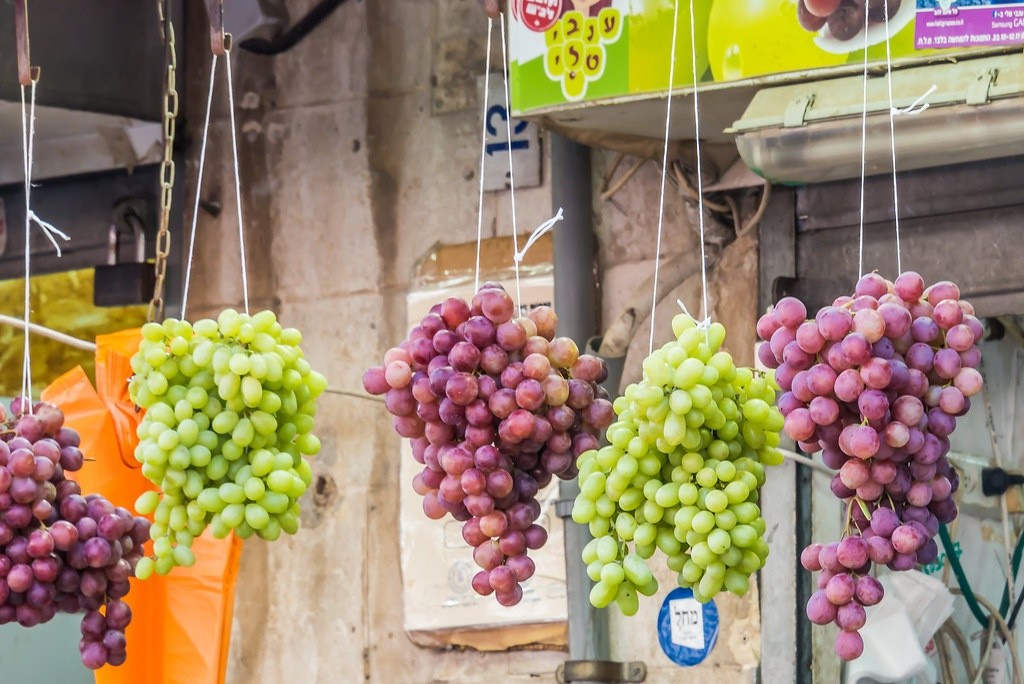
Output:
[362,281,613,606]
[128,307,326,579]
[796,0,901,41]
[572,313,784,616]
[756,271,985,662]
[0,397,151,670]
